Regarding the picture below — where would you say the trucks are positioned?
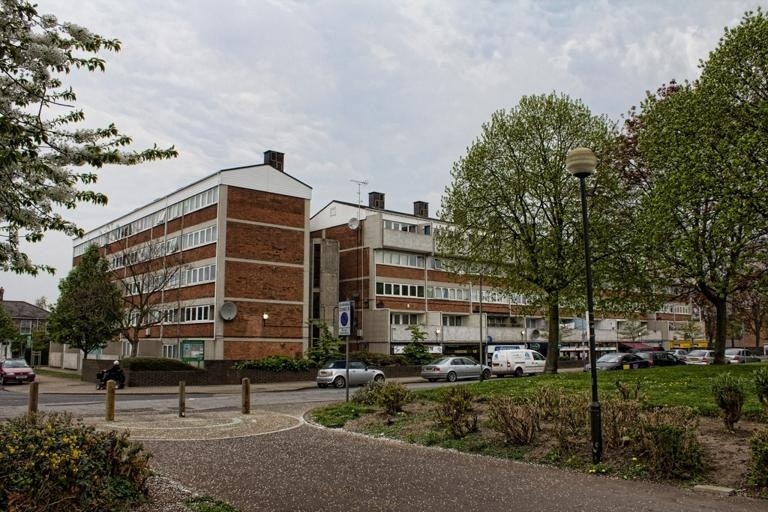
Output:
[491,350,548,377]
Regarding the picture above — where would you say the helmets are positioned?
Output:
[113,360,119,366]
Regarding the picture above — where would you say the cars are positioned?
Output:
[421,357,493,383]
[1,358,37,384]
[581,345,759,375]
[316,359,386,387]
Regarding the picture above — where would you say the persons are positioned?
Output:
[101,360,121,390]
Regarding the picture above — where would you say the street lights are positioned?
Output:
[563,143,607,465]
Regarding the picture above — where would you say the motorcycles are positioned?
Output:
[95,369,125,390]
